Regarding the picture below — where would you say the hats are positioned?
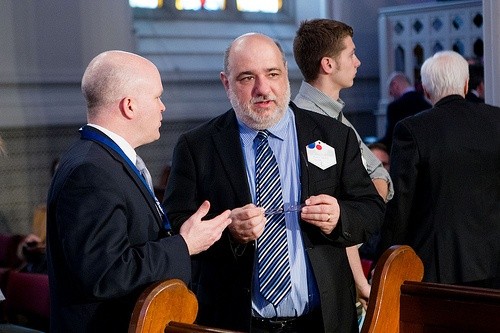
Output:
[255,130,293,308]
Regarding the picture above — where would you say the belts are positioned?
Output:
[251,311,298,332]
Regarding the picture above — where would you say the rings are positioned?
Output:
[327,214,330,222]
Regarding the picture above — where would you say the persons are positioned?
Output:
[391,50,500,288]
[367,59,486,176]
[0,235,51,275]
[161,32,388,333]
[292,18,395,312]
[44,49,232,333]
